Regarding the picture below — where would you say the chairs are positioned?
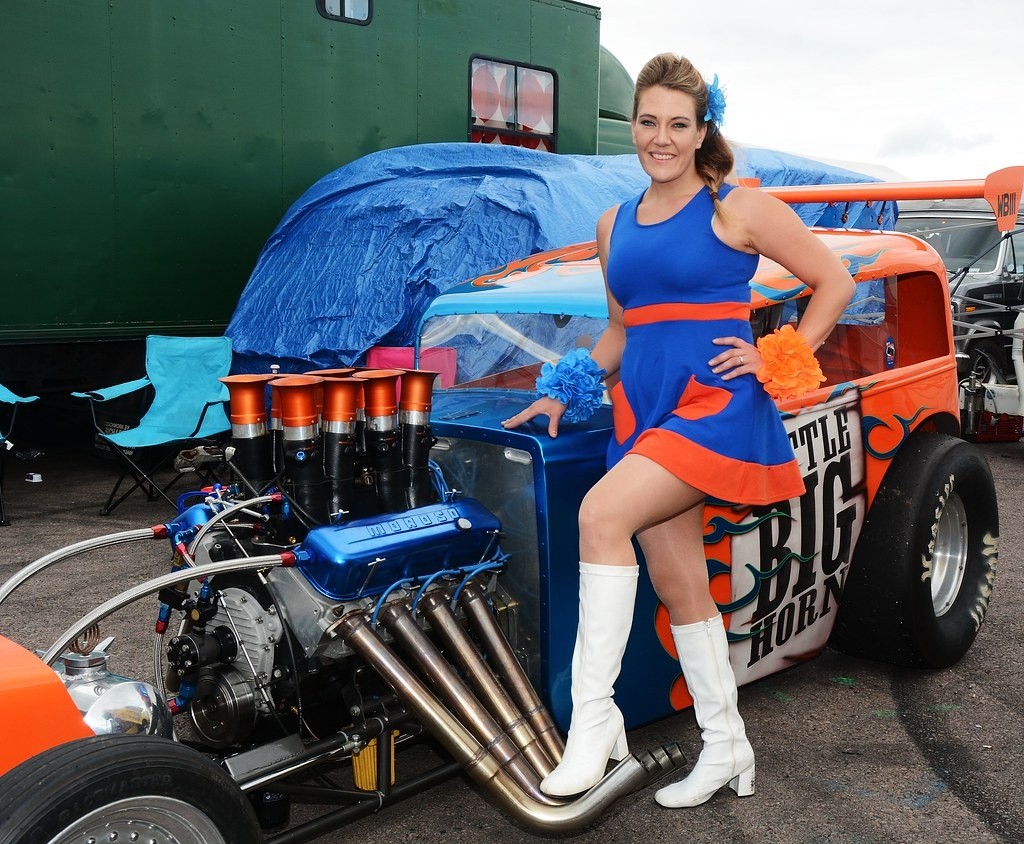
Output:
[0,383,41,526]
[70,333,233,516]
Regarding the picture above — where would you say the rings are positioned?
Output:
[738,356,744,365]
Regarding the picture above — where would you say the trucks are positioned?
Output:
[0,0,638,447]
[890,200,1024,427]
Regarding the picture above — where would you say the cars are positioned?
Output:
[1,166,1023,844]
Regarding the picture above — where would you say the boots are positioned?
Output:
[654,613,755,808]
[540,561,639,797]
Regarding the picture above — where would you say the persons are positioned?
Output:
[500,50,859,811]
